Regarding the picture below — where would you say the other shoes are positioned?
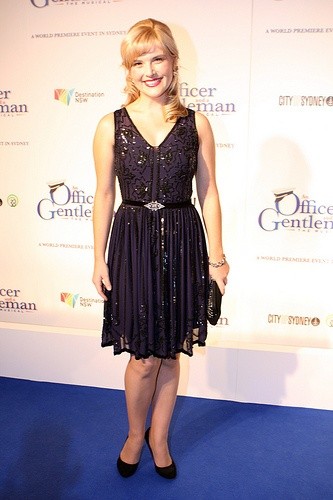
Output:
[144,426,176,480]
[117,435,143,478]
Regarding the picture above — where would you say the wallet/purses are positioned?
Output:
[208,280,222,325]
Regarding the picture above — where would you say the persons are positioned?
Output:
[92,19,229,480]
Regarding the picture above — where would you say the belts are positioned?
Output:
[123,199,191,211]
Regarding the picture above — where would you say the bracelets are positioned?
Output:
[207,254,226,268]
